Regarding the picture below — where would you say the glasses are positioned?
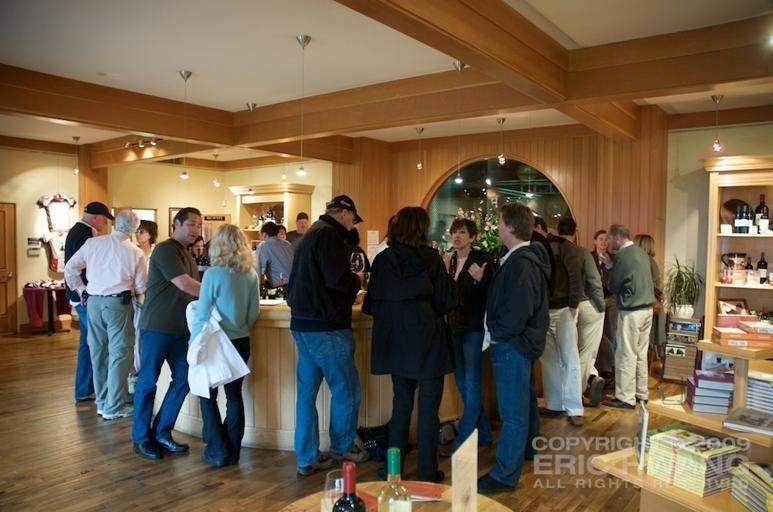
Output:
[348,211,358,224]
[135,230,149,233]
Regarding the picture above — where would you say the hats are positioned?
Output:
[84,201,115,219]
[326,194,363,224]
[297,212,308,219]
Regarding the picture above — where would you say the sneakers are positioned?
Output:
[477,474,514,492]
[441,446,453,456]
[103,406,134,420]
[76,392,96,399]
[589,376,605,406]
[570,415,583,426]
[97,403,103,415]
[127,376,137,394]
[297,450,335,475]
[537,407,565,416]
[329,442,369,462]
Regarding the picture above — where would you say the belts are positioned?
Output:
[88,294,121,297]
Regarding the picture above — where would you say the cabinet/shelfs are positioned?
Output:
[697,153,773,372]
[138,298,497,453]
[228,183,315,252]
[591,338,773,512]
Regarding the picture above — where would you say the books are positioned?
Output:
[712,314,773,348]
[633,400,650,470]
[665,320,702,358]
[722,408,773,436]
[747,368,772,412]
[686,370,735,415]
[730,461,772,512]
[647,428,742,496]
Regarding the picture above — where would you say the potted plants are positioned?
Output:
[664,258,703,319]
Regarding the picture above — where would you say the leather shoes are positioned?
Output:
[602,398,635,409]
[636,397,648,404]
[203,446,225,467]
[156,435,188,453]
[377,468,387,479]
[432,470,444,482]
[134,441,162,459]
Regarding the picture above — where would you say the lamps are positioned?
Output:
[138,139,144,147]
[178,71,192,180]
[124,142,132,148]
[246,102,256,196]
[212,153,219,188]
[496,118,507,165]
[415,128,425,171]
[150,137,157,146]
[453,60,465,185]
[72,136,80,175]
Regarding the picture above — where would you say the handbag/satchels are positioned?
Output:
[358,423,411,460]
[439,418,460,445]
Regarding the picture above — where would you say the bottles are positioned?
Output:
[741,205,748,233]
[275,277,287,300]
[747,207,754,233]
[734,206,742,233]
[378,448,412,512]
[330,462,366,512]
[196,248,202,281]
[258,273,267,299]
[757,252,768,284]
[755,193,769,234]
[188,246,194,260]
[201,248,210,277]
[745,257,754,270]
[759,206,770,233]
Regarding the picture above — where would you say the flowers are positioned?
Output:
[711,94,723,154]
[294,35,310,180]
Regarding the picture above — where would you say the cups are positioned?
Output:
[321,469,344,512]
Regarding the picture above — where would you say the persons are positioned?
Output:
[286,195,371,477]
[533,216,585,426]
[599,224,655,410]
[557,217,606,407]
[370,214,396,265]
[438,218,493,458]
[478,204,551,493]
[185,224,260,467]
[65,209,146,420]
[132,220,158,375]
[65,202,115,403]
[362,206,458,482]
[286,212,309,245]
[275,224,287,240]
[189,236,204,259]
[132,207,202,459]
[633,234,662,347]
[255,222,295,298]
[591,229,617,389]
